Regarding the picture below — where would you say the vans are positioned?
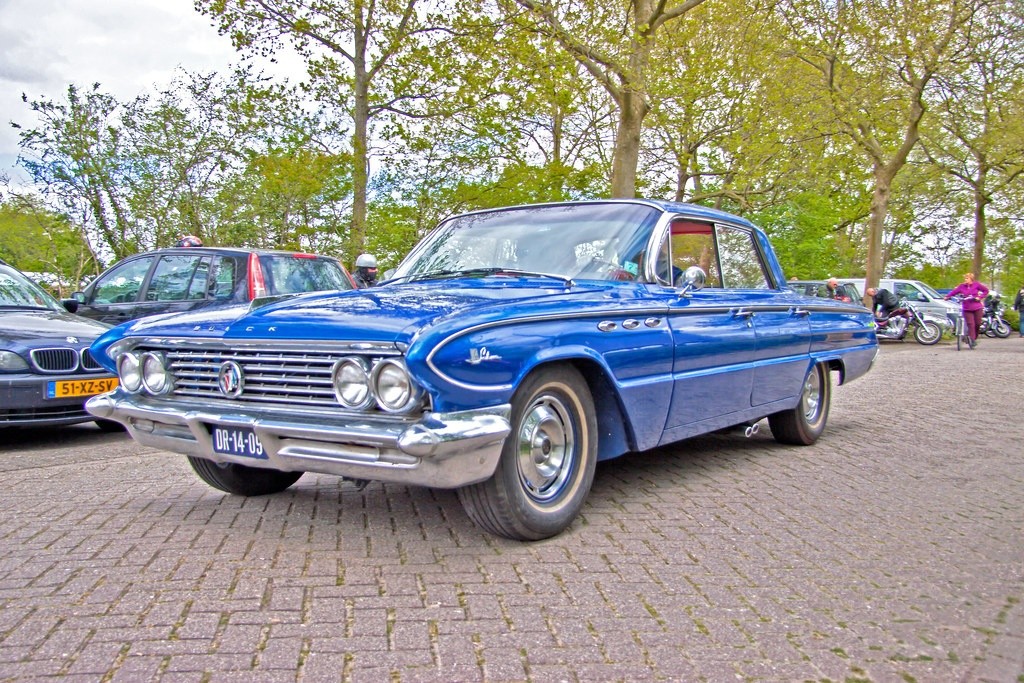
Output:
[823,278,960,322]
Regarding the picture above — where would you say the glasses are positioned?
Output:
[368,267,377,270]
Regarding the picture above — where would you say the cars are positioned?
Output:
[91,201,884,541]
[66,245,364,332]
[0,259,128,434]
[753,280,864,307]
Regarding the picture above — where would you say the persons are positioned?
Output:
[790,277,838,299]
[944,272,988,347]
[866,288,901,318]
[351,253,379,289]
[613,223,683,287]
[1013,286,1024,338]
[514,235,539,268]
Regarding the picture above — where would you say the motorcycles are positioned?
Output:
[869,297,942,345]
[974,294,1012,339]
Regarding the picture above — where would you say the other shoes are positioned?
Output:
[972,339,977,347]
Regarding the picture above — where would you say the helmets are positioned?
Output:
[179,236,202,249]
[356,254,378,267]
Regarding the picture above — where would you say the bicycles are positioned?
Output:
[949,295,978,351]
